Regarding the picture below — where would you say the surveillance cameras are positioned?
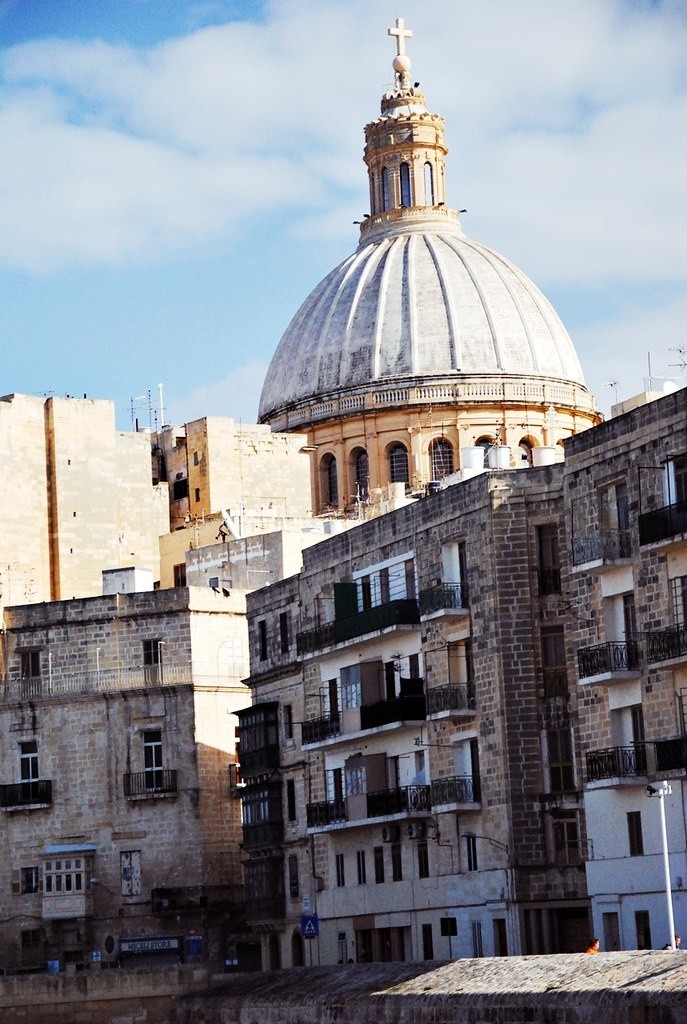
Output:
[646,786,656,793]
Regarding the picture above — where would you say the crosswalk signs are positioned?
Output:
[300,915,318,936]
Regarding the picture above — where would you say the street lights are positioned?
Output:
[645,780,677,951]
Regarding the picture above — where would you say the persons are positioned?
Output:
[662,935,681,950]
[587,937,600,953]
